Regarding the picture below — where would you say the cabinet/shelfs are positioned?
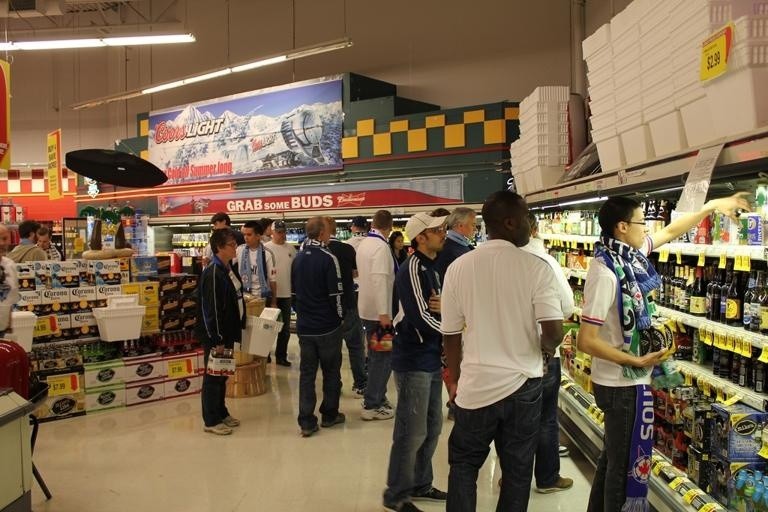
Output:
[522,128,767,512]
[149,203,488,333]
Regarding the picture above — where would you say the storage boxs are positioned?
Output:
[509,86,571,196]
[0,248,206,425]
[242,307,283,359]
[580,0,767,176]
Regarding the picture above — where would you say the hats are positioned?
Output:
[270,220,288,231]
[406,213,447,240]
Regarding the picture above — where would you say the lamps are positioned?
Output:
[0,0,200,52]
[68,0,354,111]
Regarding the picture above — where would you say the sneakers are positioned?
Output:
[302,424,318,436]
[321,413,345,426]
[559,445,569,456]
[383,501,424,512]
[411,485,448,502]
[352,385,396,420]
[222,416,240,426]
[276,358,291,366]
[536,478,573,494]
[204,423,232,435]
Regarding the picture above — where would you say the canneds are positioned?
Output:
[639,317,676,360]
[738,212,764,246]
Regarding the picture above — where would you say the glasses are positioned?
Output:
[423,222,448,233]
[226,242,238,248]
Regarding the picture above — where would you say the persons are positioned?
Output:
[382,189,575,511]
[198,191,480,437]
[576,191,755,512]
[4,219,48,263]
[34,226,66,262]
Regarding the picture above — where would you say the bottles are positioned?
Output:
[52,272,61,289]
[286,227,307,244]
[389,227,408,245]
[173,247,202,257]
[0,196,24,225]
[53,219,77,259]
[95,271,104,285]
[533,179,768,512]
[35,272,42,285]
[29,341,81,362]
[336,227,352,241]
[82,331,201,362]
[211,347,233,359]
[170,232,209,243]
[130,209,148,256]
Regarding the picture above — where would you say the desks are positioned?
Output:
[0,384,37,512]
